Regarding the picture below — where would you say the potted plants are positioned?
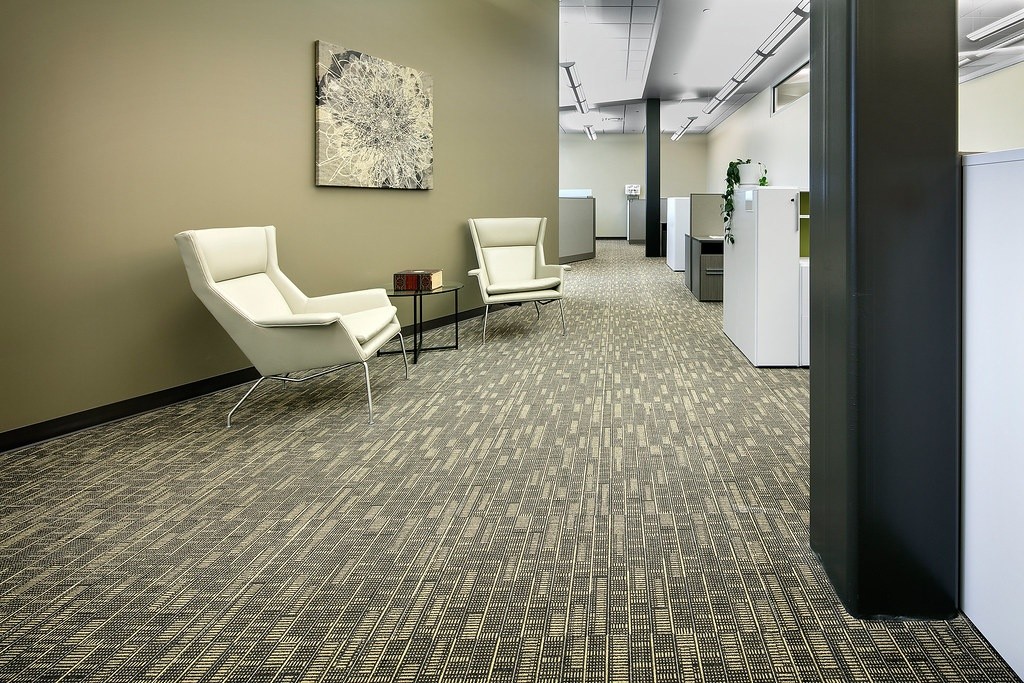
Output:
[720,157,769,245]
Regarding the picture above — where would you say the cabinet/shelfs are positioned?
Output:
[722,186,811,368]
[666,197,690,272]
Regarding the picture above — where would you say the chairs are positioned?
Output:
[468,217,566,345]
[174,226,408,428]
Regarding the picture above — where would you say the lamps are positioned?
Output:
[583,125,597,141]
[958,29,1024,68]
[966,8,1024,42]
[701,0,810,114]
[559,62,590,114]
[671,117,698,142]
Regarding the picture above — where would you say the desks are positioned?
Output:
[685,193,724,301]
[374,283,465,364]
